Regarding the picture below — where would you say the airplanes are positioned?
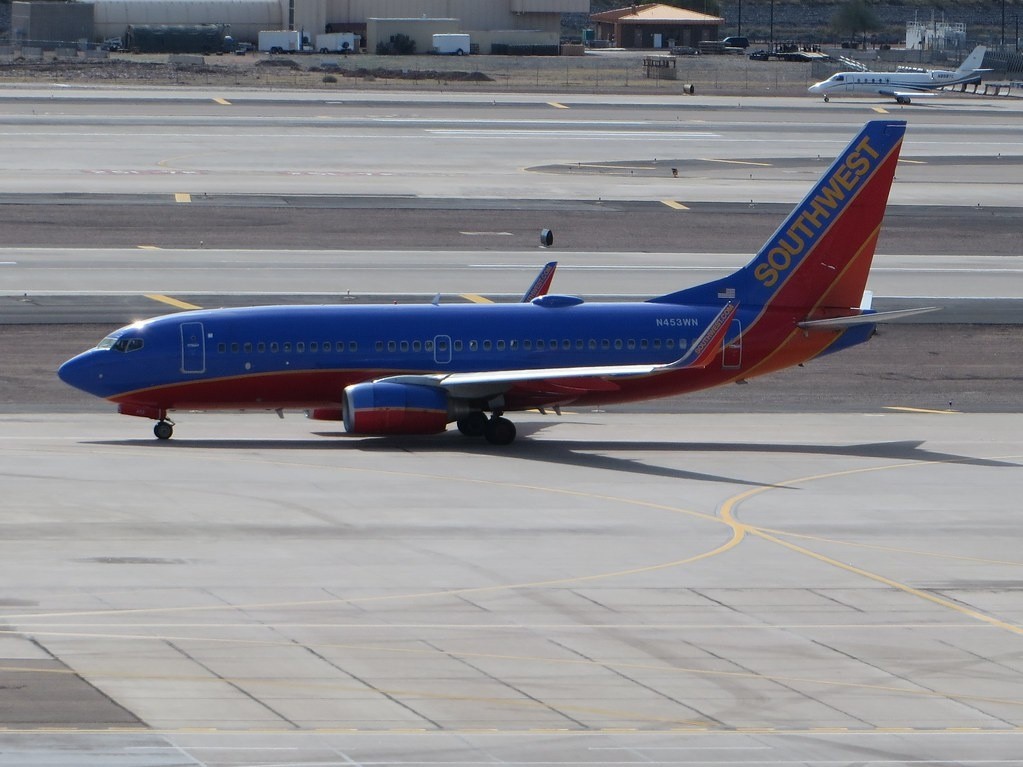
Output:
[808,43,995,106]
[51,121,945,443]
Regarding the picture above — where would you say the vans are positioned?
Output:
[722,37,750,48]
